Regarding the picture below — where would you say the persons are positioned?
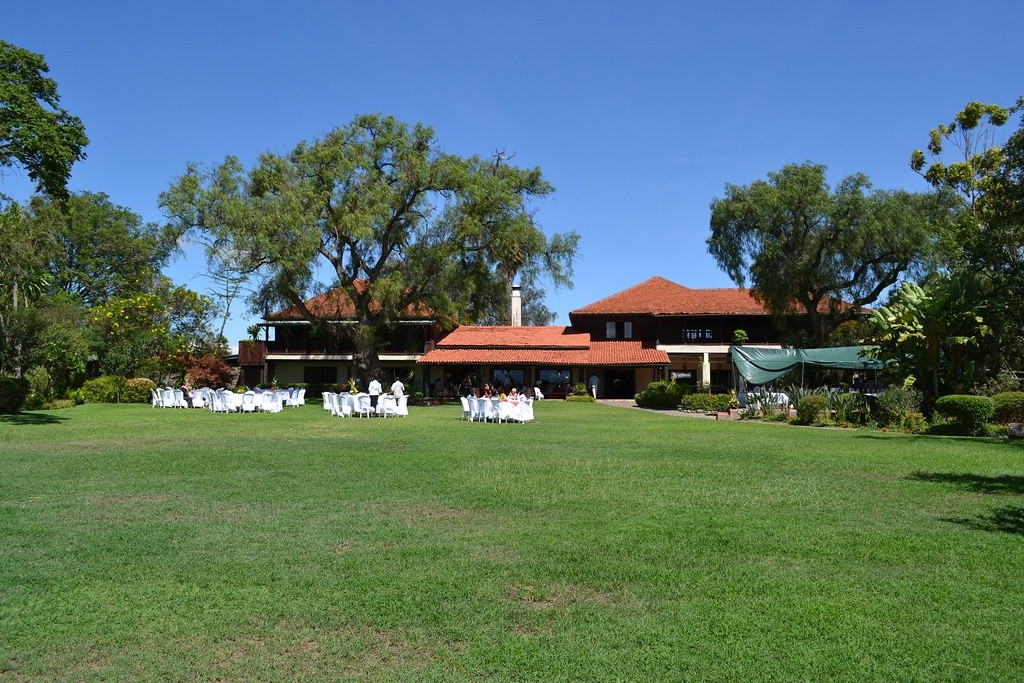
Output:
[368,376,382,412]
[588,373,599,399]
[509,388,517,397]
[391,377,405,406]
[485,384,489,395]
[504,374,511,396]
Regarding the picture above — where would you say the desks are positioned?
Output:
[738,393,789,409]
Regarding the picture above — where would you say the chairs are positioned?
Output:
[321,391,409,419]
[188,386,306,413]
[150,386,188,409]
[460,387,544,425]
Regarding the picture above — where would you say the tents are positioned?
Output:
[727,346,897,388]
